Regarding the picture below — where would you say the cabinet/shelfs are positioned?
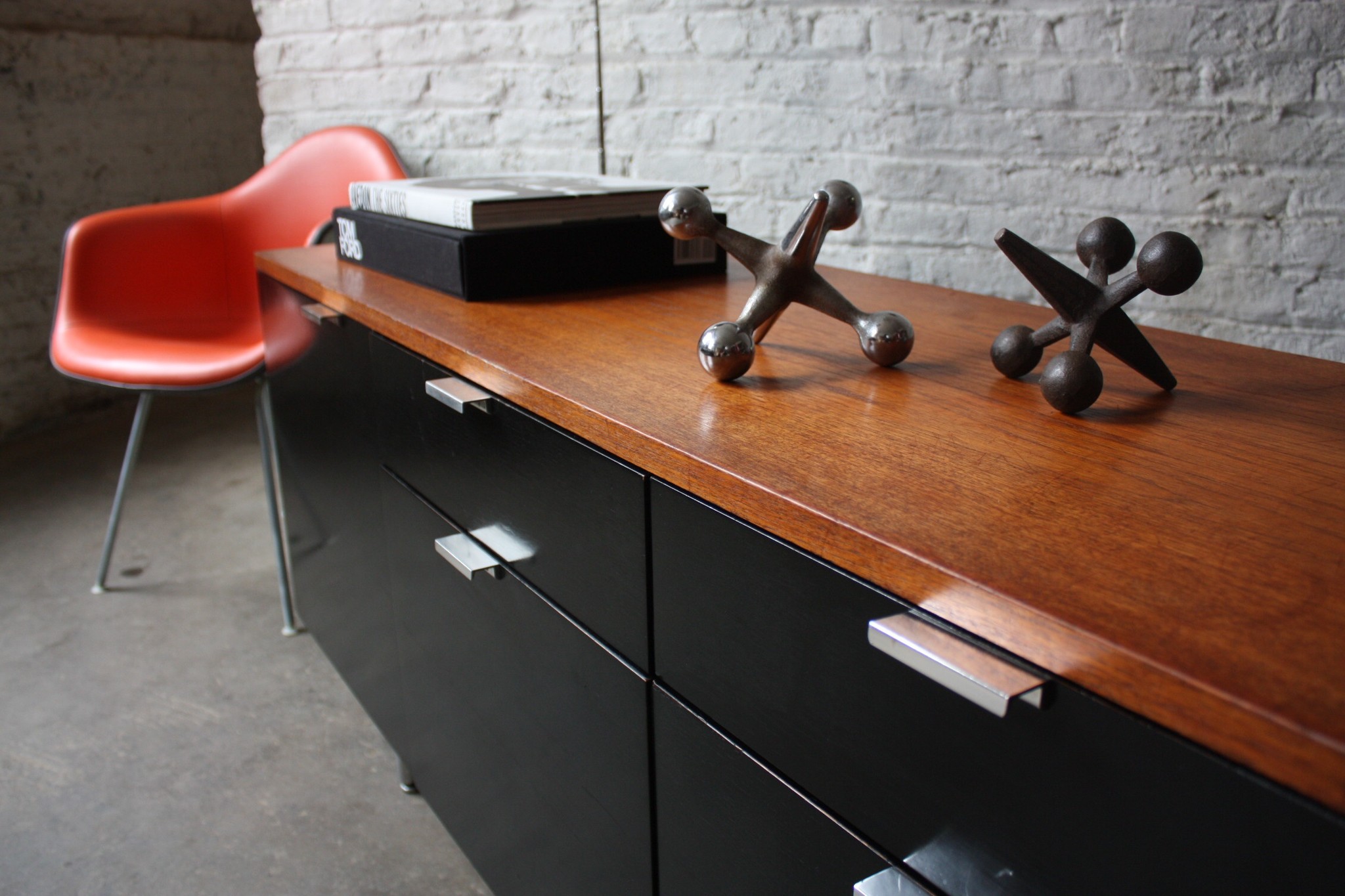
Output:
[257,241,1345,896]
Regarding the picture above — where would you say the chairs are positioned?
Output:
[48,126,408,637]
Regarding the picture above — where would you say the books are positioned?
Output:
[349,173,708,231]
